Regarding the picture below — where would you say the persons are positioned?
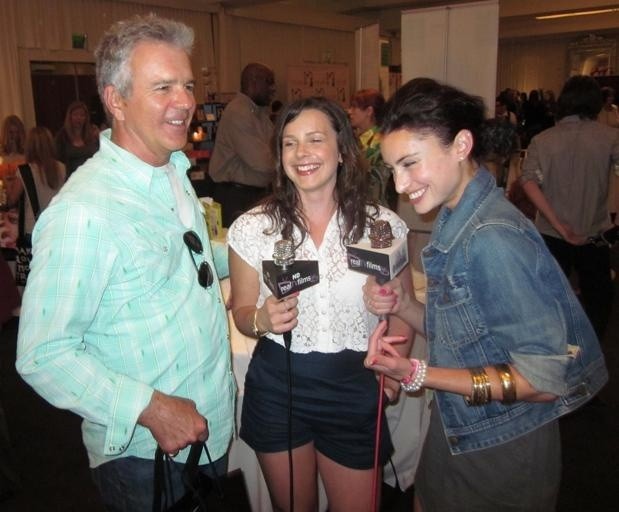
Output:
[2,103,112,286]
[226,95,416,511]
[347,89,399,214]
[210,64,285,226]
[14,8,238,512]
[497,74,618,317]
[365,75,609,512]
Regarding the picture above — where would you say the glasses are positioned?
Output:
[183,231,213,287]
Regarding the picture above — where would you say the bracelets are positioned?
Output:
[401,356,429,390]
[247,309,270,339]
[496,365,518,407]
[463,362,493,407]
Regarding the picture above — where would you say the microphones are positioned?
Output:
[346,221,409,336]
[264,241,318,348]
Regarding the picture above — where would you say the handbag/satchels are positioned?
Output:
[14,234,32,288]
[152,441,252,512]
